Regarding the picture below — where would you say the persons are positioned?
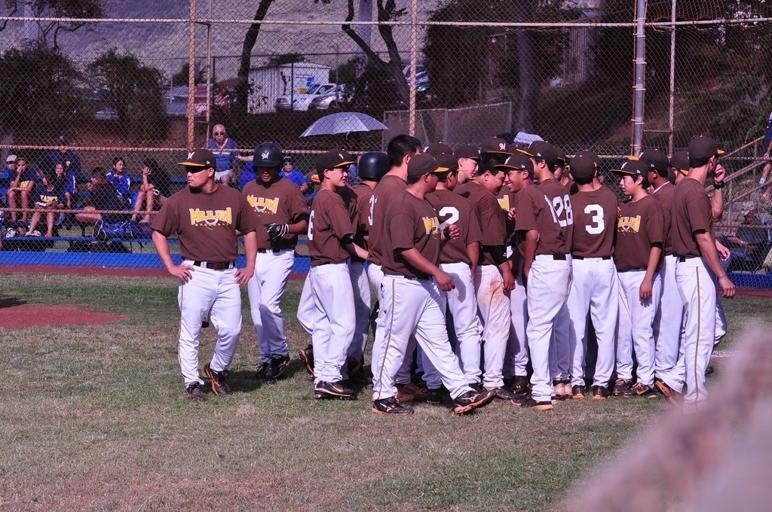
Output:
[150,149,257,397]
[295,132,736,416]
[0,133,137,239]
[281,154,308,195]
[131,158,172,223]
[206,124,237,187]
[240,143,308,382]
[758,109,772,190]
[717,200,769,272]
[236,155,257,191]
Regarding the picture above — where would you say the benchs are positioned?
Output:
[1,173,189,254]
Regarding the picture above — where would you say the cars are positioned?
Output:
[310,86,355,109]
[550,75,564,86]
[401,63,432,93]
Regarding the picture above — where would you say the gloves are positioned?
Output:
[266,222,289,241]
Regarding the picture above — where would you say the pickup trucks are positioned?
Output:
[277,83,337,110]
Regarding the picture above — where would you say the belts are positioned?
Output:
[573,256,611,260]
[553,255,566,260]
[258,248,281,254]
[680,256,686,263]
[182,257,236,271]
[617,266,647,272]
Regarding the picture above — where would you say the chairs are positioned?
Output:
[728,229,772,274]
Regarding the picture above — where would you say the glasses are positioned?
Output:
[186,166,204,173]
[214,132,223,136]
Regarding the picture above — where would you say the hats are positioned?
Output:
[408,154,450,177]
[516,141,559,164]
[5,154,17,163]
[481,138,513,156]
[317,150,357,171]
[426,144,459,174]
[455,146,481,159]
[688,137,726,160]
[177,149,216,172]
[557,147,691,177]
[496,154,535,178]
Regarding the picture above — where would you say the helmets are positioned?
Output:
[359,152,390,180]
[253,141,285,169]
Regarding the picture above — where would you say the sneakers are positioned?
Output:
[495,366,714,410]
[5,228,42,240]
[454,390,498,415]
[372,397,415,417]
[186,381,206,401]
[256,344,442,404]
[204,363,232,398]
